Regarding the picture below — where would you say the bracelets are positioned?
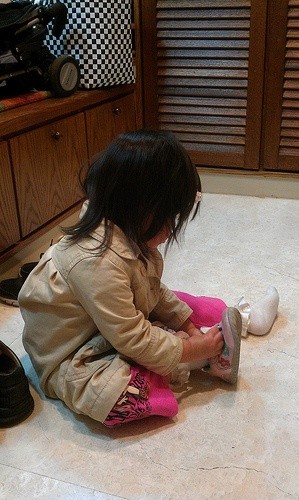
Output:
[185,327,196,332]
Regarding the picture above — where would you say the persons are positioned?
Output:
[17,129,278,427]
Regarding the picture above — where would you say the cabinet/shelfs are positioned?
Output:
[0,140,22,265]
[8,113,96,238]
[85,91,139,164]
[141,0,299,181]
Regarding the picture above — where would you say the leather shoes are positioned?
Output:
[0,339,34,428]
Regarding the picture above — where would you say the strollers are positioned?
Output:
[0,0,81,97]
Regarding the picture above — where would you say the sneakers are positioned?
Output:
[0,238,53,307]
[201,307,242,384]
